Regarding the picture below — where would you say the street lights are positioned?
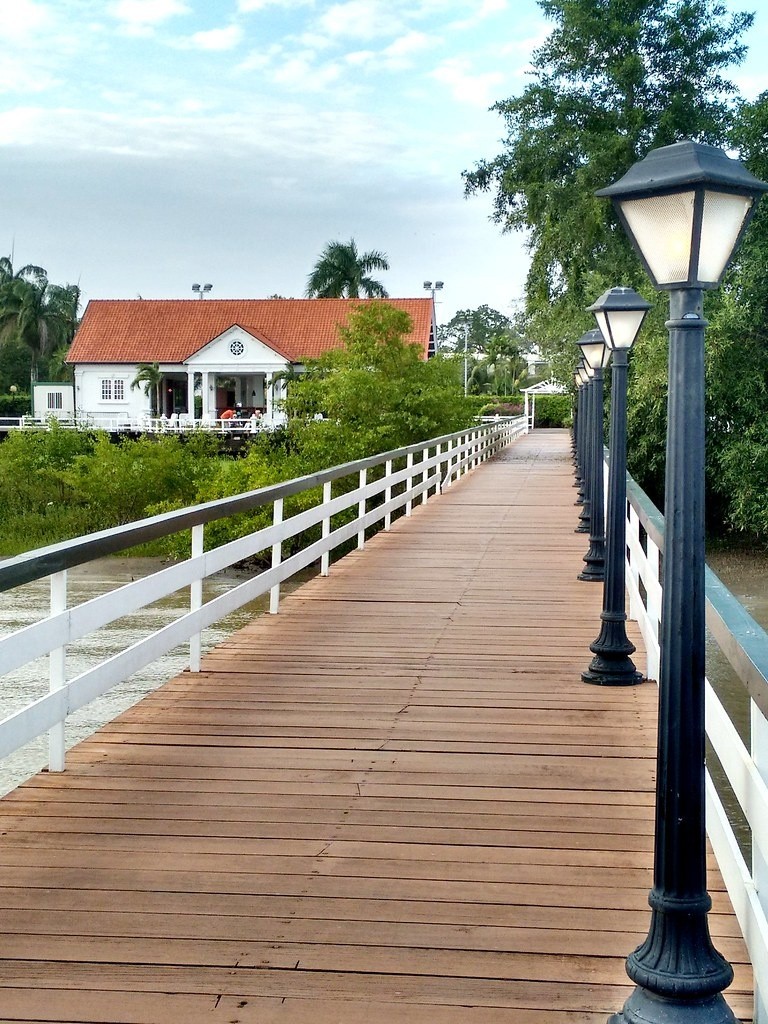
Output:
[458,314,472,398]
[9,386,17,415]
[423,281,444,359]
[191,283,214,300]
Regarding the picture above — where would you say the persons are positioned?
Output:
[220,410,236,422]
[247,410,261,431]
